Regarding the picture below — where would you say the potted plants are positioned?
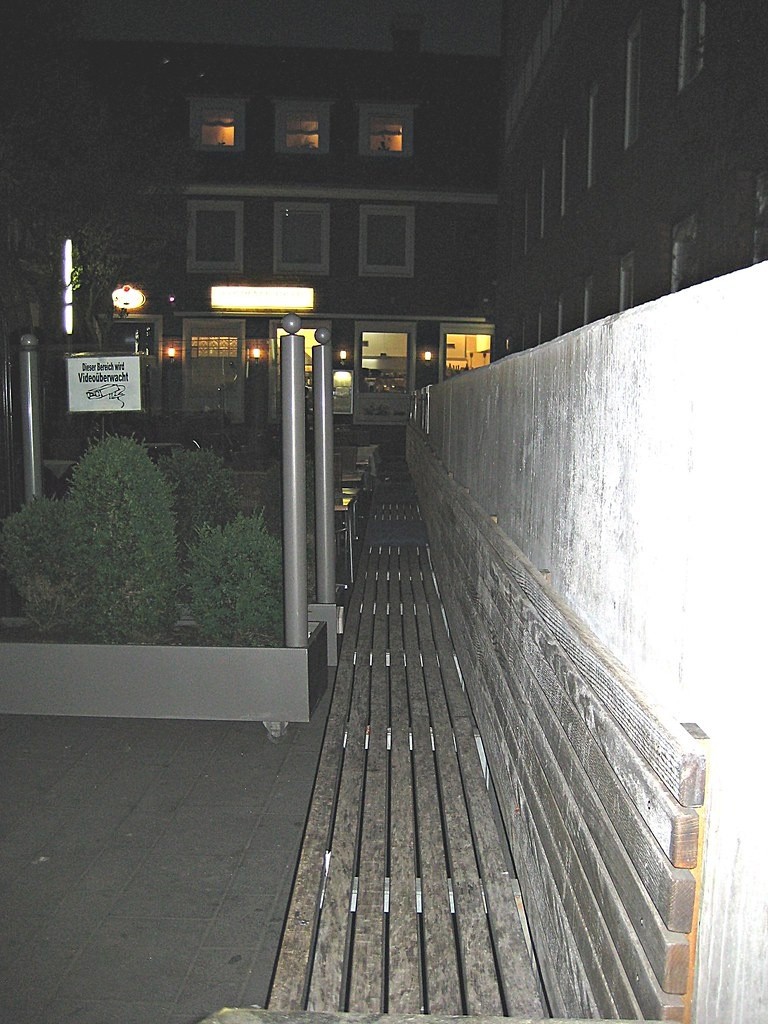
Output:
[0,432,349,723]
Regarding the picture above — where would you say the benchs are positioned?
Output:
[200,415,710,1023]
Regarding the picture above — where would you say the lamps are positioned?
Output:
[254,348,260,365]
[425,351,431,368]
[168,347,174,364]
[340,350,347,366]
